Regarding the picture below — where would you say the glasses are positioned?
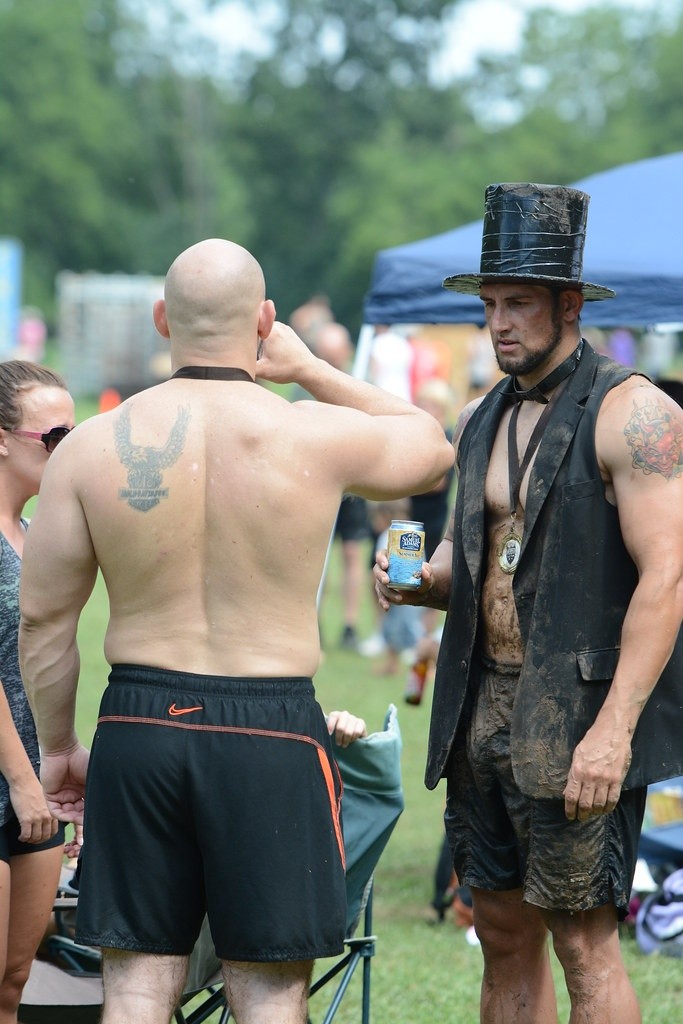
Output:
[7,423,76,455]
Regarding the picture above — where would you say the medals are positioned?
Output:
[495,533,522,575]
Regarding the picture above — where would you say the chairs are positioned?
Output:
[38,699,405,1024]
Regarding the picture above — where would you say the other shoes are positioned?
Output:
[341,625,379,658]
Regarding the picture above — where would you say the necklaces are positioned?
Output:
[169,366,255,383]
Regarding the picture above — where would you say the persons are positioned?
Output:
[0,360,75,1024]
[16,239,456,1024]
[286,303,501,678]
[44,711,368,938]
[372,182,683,1024]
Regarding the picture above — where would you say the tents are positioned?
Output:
[318,153,683,607]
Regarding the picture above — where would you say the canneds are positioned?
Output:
[388,519,426,590]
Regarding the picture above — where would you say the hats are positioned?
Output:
[443,180,618,302]
